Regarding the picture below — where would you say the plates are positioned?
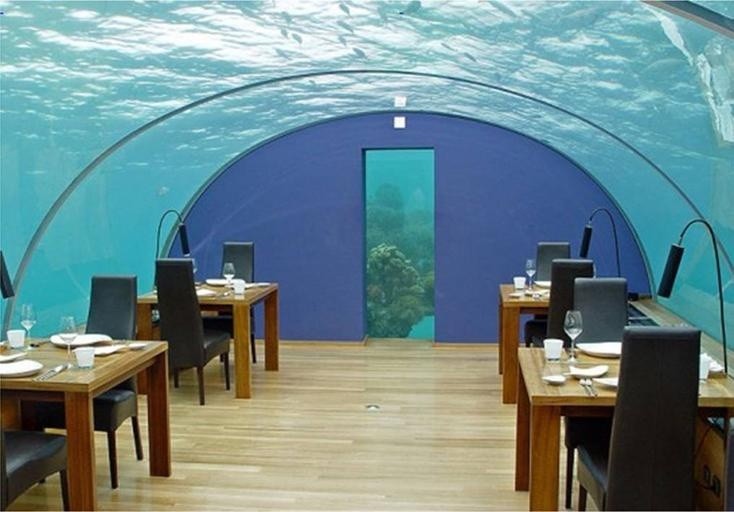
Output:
[205,278,227,286]
[48,333,113,349]
[575,341,622,358]
[0,358,43,378]
[570,365,610,380]
[591,376,620,389]
[533,280,552,288]
[72,345,127,358]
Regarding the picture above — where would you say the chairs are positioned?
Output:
[576,328,701,510]
[525,257,596,346]
[217,240,257,364]
[0,421,72,510]
[35,275,143,488]
[533,240,573,322]
[155,258,231,404]
[560,275,628,510]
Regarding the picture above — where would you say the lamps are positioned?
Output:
[0,251,15,298]
[580,207,622,278]
[657,219,730,377]
[150,209,192,294]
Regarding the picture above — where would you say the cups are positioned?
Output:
[222,262,236,289]
[7,330,26,349]
[73,346,95,369]
[233,278,246,295]
[513,276,526,294]
[58,315,78,369]
[543,339,564,364]
[563,309,584,365]
[525,257,537,291]
[21,302,38,353]
